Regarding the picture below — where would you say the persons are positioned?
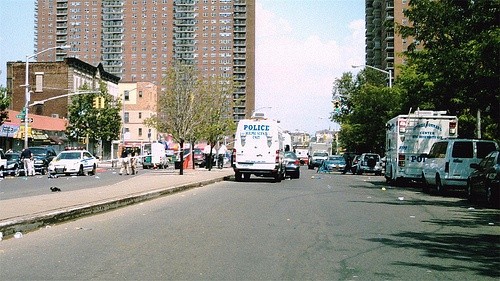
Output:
[20,147,34,176]
[341,156,352,175]
[119,145,139,175]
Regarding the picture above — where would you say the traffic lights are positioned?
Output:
[93,97,99,108]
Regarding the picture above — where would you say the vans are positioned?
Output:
[282,132,294,153]
[230,119,290,183]
[420,138,498,195]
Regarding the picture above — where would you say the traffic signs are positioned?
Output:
[21,118,33,123]
[3,127,13,131]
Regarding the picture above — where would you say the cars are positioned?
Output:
[465,150,500,207]
[281,152,301,179]
[47,149,98,177]
[4,153,22,177]
[321,155,346,173]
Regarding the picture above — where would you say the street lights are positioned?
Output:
[351,64,392,91]
[24,45,72,178]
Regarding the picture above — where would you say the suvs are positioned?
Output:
[183,148,205,167]
[19,146,57,176]
[0,148,8,178]
[351,153,386,177]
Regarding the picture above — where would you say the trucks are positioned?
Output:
[294,148,309,166]
[384,107,458,186]
[307,142,333,170]
